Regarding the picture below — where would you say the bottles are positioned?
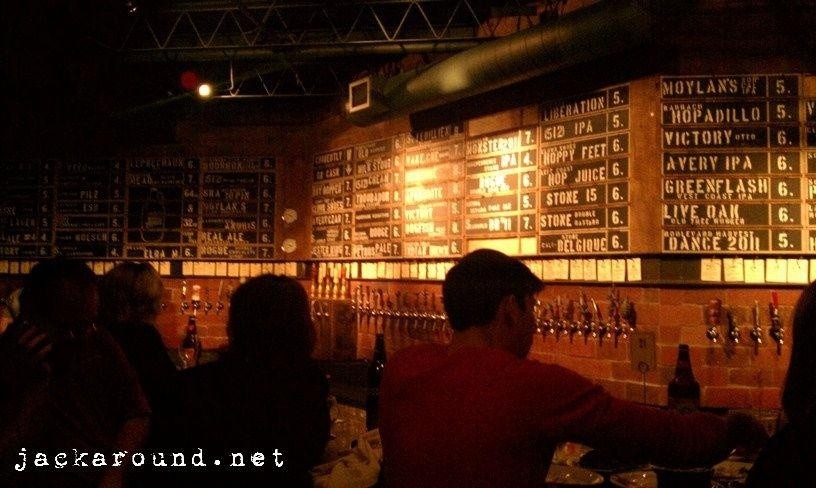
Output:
[178,313,200,369]
[668,342,700,411]
[367,332,387,393]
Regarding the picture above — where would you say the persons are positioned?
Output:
[375,248,769,488]
[743,279,816,487]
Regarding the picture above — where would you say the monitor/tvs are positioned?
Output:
[348,76,371,113]
